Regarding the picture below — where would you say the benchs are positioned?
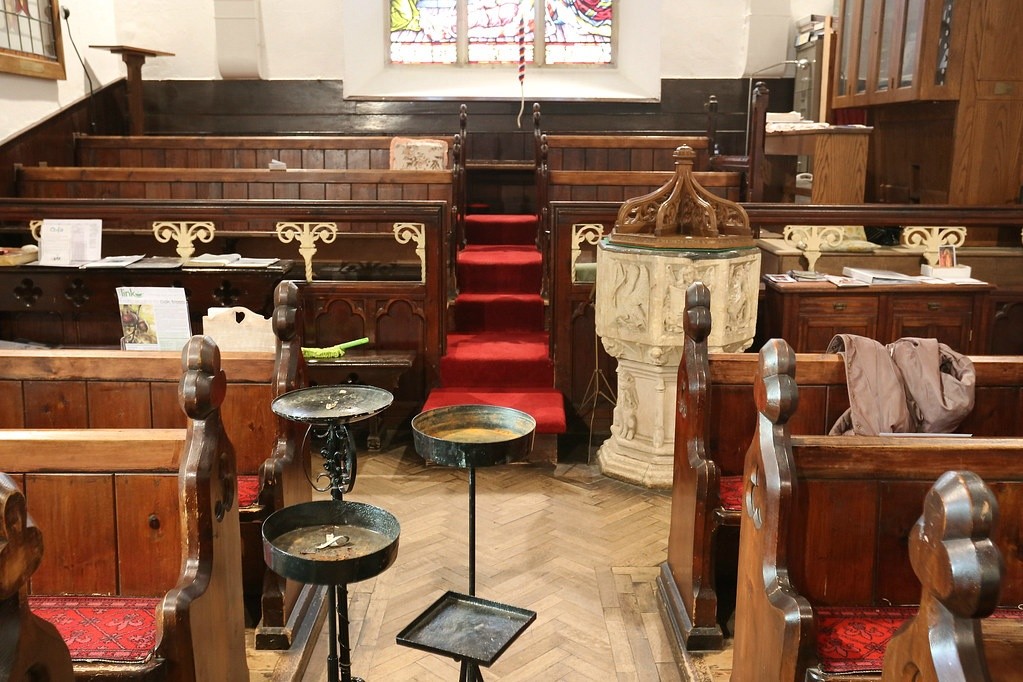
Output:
[535,80,1023,680]
[0,100,463,682]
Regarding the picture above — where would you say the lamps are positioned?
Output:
[745,59,809,157]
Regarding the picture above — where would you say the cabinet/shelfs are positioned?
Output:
[0,262,290,347]
[772,270,998,357]
[832,0,1023,207]
